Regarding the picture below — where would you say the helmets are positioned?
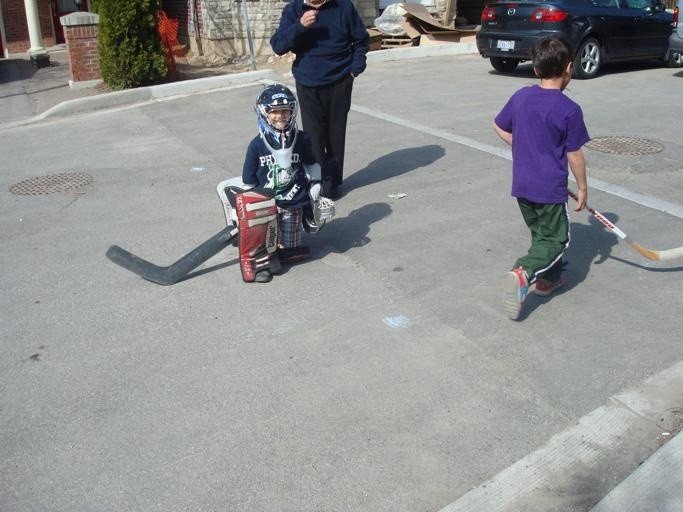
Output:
[257,84,296,118]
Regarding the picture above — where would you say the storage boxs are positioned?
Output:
[366,29,383,51]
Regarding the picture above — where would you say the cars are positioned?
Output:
[475,0,682,79]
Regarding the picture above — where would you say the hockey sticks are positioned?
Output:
[567,190,683,261]
[108,225,241,286]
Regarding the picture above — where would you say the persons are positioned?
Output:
[240,83,323,284]
[492,38,591,319]
[270,1,370,199]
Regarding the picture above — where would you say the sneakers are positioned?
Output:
[535,274,567,297]
[505,268,530,320]
[253,267,273,282]
